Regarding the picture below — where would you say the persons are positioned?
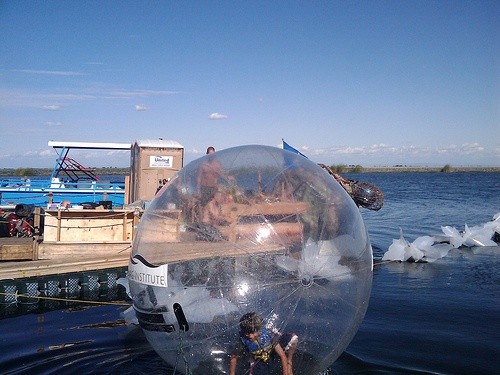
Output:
[8,204,33,238]
[226,312,298,375]
[186,147,237,242]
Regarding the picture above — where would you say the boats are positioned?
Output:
[0,139,310,335]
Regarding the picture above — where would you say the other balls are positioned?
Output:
[126,144,375,375]
